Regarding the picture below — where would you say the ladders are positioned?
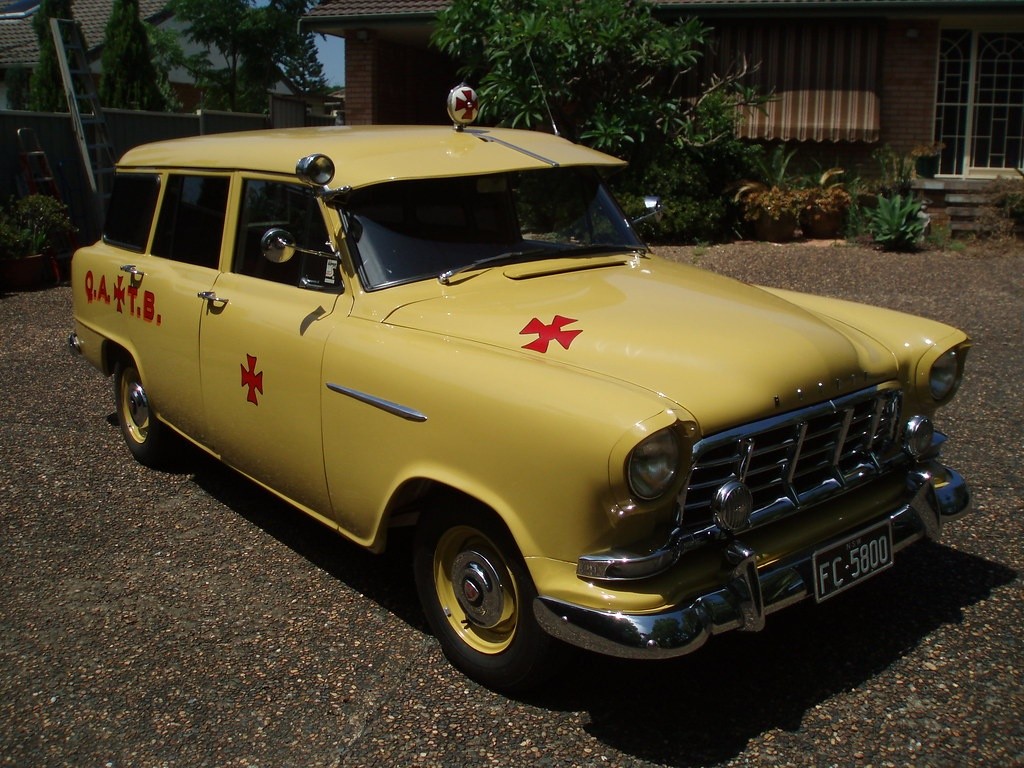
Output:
[46,15,132,227]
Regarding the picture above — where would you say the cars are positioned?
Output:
[70,85,975,692]
